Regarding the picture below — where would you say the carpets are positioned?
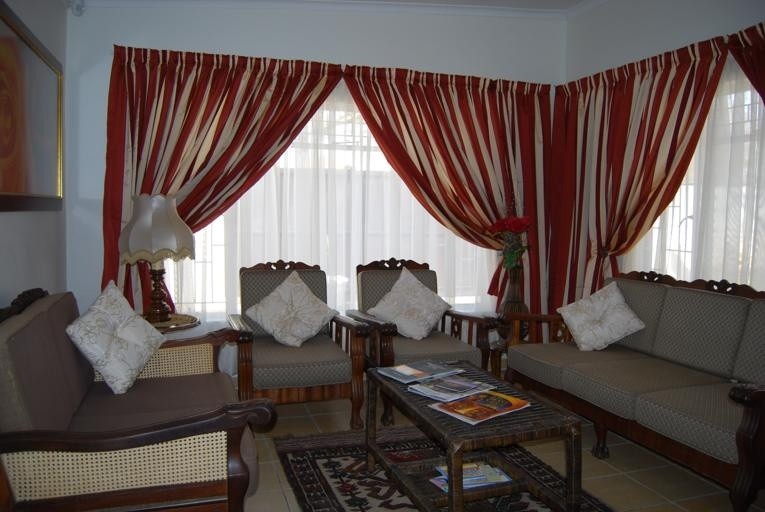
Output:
[269,424,619,512]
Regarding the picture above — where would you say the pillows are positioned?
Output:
[365,265,453,340]
[557,280,647,353]
[64,279,167,396]
[245,268,339,348]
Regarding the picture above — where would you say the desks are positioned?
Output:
[138,311,201,333]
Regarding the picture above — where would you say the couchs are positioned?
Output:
[502,270,765,512]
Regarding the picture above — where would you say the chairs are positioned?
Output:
[345,257,499,425]
[226,258,370,430]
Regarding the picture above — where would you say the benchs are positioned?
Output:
[2,259,277,512]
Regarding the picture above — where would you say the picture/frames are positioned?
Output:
[0,0,64,213]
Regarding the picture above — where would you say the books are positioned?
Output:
[375,359,465,386]
[427,387,532,427]
[407,373,501,404]
